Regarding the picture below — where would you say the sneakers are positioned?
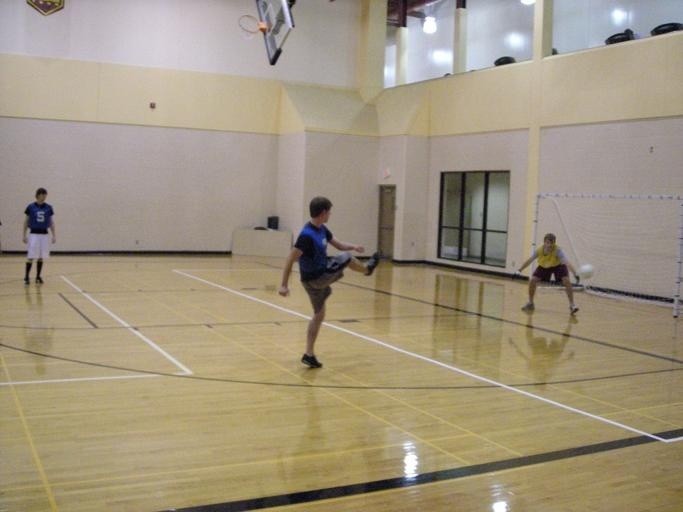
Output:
[23,277,41,283]
[521,303,535,310]
[300,353,322,368]
[364,251,378,276]
[570,304,579,312]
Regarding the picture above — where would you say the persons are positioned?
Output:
[21,187,57,286]
[19,282,55,376]
[279,195,381,368]
[506,309,579,392]
[511,232,579,315]
[281,359,368,499]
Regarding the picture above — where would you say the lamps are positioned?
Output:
[423,0,437,34]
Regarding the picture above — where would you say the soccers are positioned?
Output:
[579,265,594,280]
